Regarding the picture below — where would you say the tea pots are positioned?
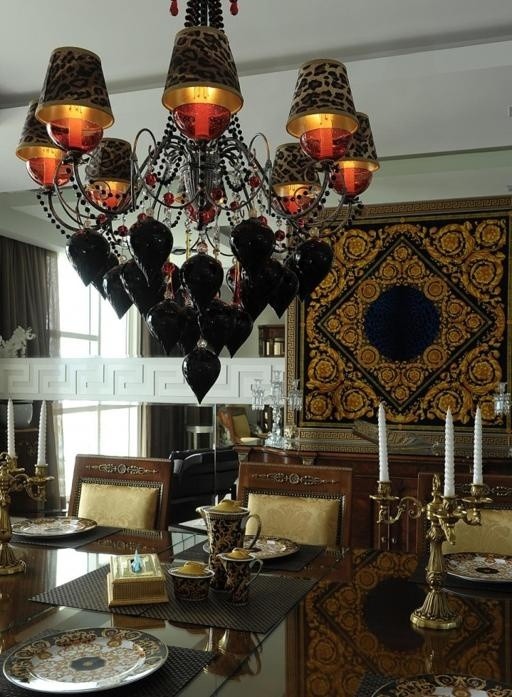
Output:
[215,546,265,607]
[201,626,263,683]
[194,500,260,575]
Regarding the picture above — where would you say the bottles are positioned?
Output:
[266,337,285,356]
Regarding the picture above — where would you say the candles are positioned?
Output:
[473,404,485,488]
[6,394,17,457]
[376,403,391,481]
[36,399,48,466]
[445,405,457,498]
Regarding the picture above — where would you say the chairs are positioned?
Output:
[413,471,511,559]
[66,452,176,532]
[235,460,355,549]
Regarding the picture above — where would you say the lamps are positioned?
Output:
[11,0,381,407]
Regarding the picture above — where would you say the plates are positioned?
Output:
[441,576,511,601]
[204,534,300,561]
[441,550,511,583]
[3,626,169,692]
[11,517,97,540]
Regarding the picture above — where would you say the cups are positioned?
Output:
[167,566,215,600]
